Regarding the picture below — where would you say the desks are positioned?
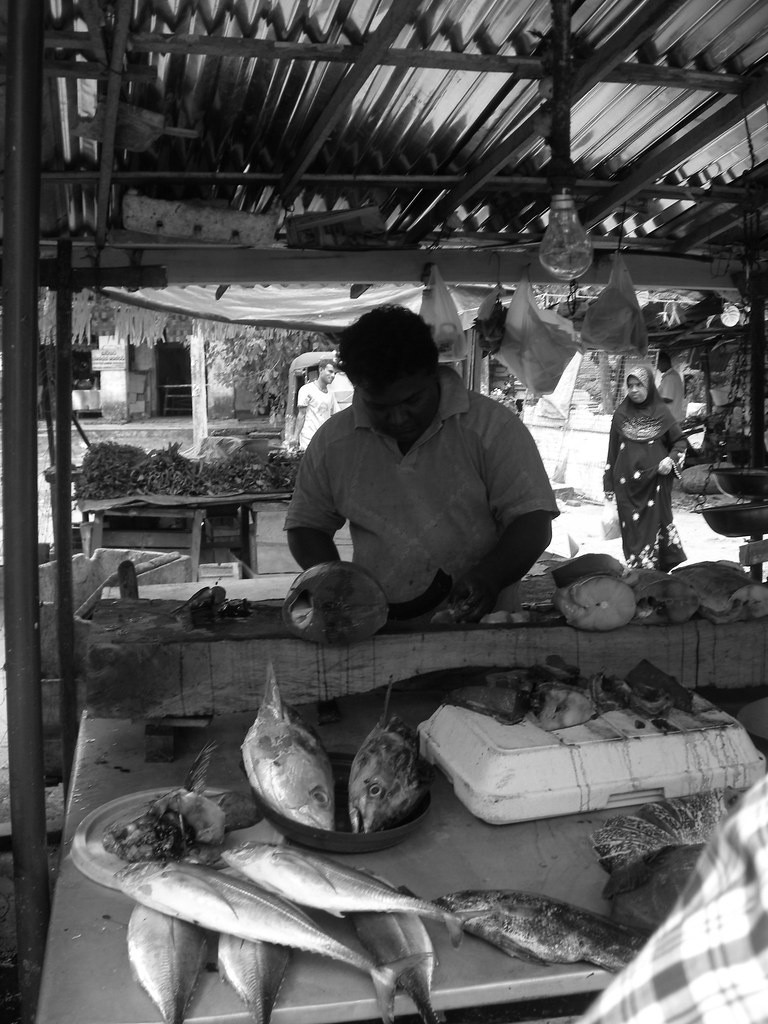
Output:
[75,480,295,583]
[159,385,192,416]
[35,575,617,1024]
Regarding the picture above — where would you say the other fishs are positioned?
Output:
[98,658,746,1024]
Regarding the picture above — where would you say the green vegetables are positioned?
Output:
[68,441,307,499]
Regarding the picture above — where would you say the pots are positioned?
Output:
[239,752,432,854]
[690,501,768,538]
[707,464,768,500]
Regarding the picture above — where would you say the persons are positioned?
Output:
[652,351,685,428]
[289,358,338,459]
[603,364,688,575]
[281,302,560,626]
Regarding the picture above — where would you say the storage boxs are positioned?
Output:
[417,690,768,826]
[205,517,240,548]
[38,547,191,779]
[199,562,243,582]
[122,195,279,244]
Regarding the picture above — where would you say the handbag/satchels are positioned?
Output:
[492,267,579,398]
[601,498,621,540]
[580,250,649,357]
[419,264,469,363]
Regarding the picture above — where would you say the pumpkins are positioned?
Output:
[550,577,637,630]
